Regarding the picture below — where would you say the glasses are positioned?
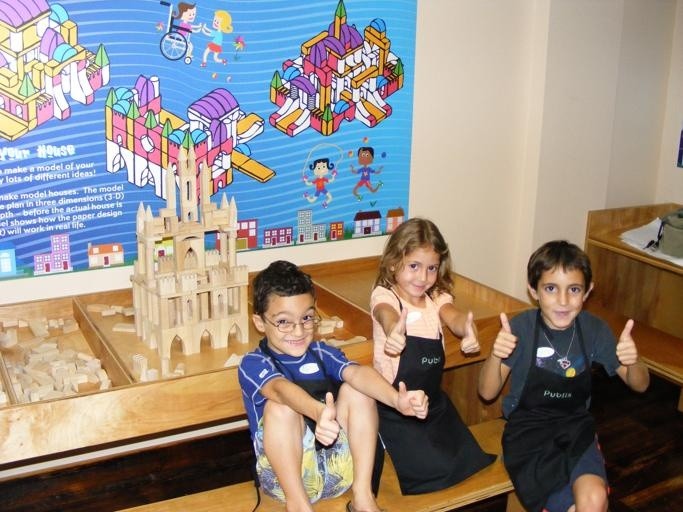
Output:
[263,311,321,333]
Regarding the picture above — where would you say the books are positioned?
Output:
[620,214,682,268]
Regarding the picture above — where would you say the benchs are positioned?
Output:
[104,416,525,512]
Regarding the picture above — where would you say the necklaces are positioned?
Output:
[541,319,577,370]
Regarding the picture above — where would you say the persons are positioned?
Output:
[477,236,653,512]
[236,260,431,511]
[363,216,481,496]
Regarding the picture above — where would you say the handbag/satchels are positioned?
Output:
[659,212,683,257]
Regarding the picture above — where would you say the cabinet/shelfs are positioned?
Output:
[582,201,683,411]
